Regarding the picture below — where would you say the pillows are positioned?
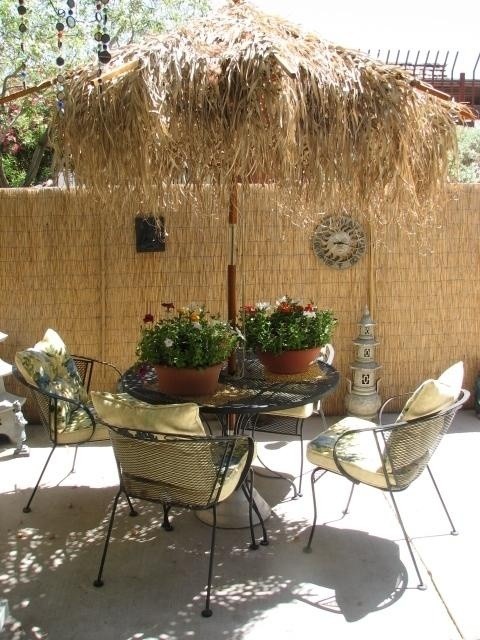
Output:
[383,361,464,473]
[15,329,86,434]
[91,391,221,490]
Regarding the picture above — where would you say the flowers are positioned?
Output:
[237,294,339,358]
[135,301,245,370]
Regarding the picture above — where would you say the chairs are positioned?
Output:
[94,418,259,617]
[303,389,471,590]
[13,354,139,517]
[241,346,335,497]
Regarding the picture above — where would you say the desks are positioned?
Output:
[121,349,340,545]
